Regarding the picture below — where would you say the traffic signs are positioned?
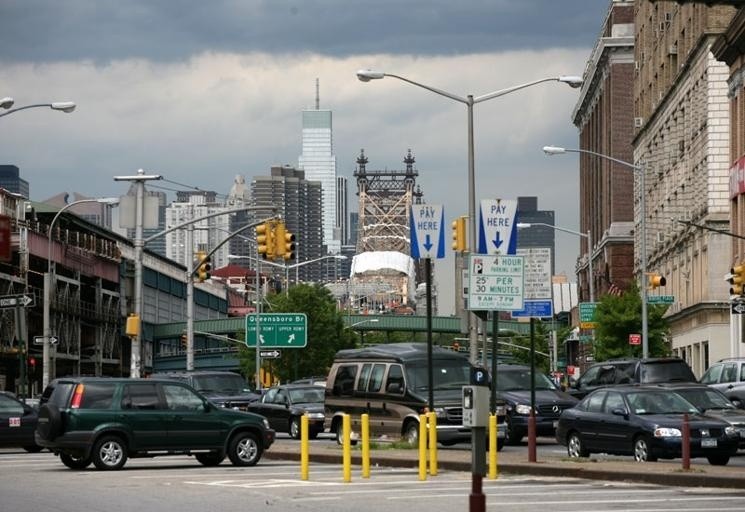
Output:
[646,295,676,305]
[408,202,445,260]
[512,301,552,318]
[33,335,60,345]
[732,303,745,314]
[258,351,281,358]
[579,301,602,322]
[580,322,599,329]
[477,198,518,255]
[245,312,307,348]
[0,292,36,308]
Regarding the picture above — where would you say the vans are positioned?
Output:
[323,341,509,453]
[568,357,699,400]
[699,356,745,408]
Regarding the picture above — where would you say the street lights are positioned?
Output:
[515,221,596,352]
[339,289,398,339]
[0,101,76,117]
[225,253,348,300]
[42,197,120,391]
[543,146,648,360]
[0,97,13,111]
[498,326,522,337]
[355,69,585,366]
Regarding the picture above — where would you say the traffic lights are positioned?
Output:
[730,262,745,294]
[453,219,466,251]
[198,250,211,281]
[254,219,295,262]
[181,334,187,347]
[27,355,39,383]
[647,272,667,289]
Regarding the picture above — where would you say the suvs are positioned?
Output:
[292,378,326,390]
[481,363,582,443]
[166,371,261,412]
[33,377,275,466]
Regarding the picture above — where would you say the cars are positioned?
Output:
[639,380,745,456]
[0,391,44,453]
[246,384,328,438]
[554,385,740,467]
